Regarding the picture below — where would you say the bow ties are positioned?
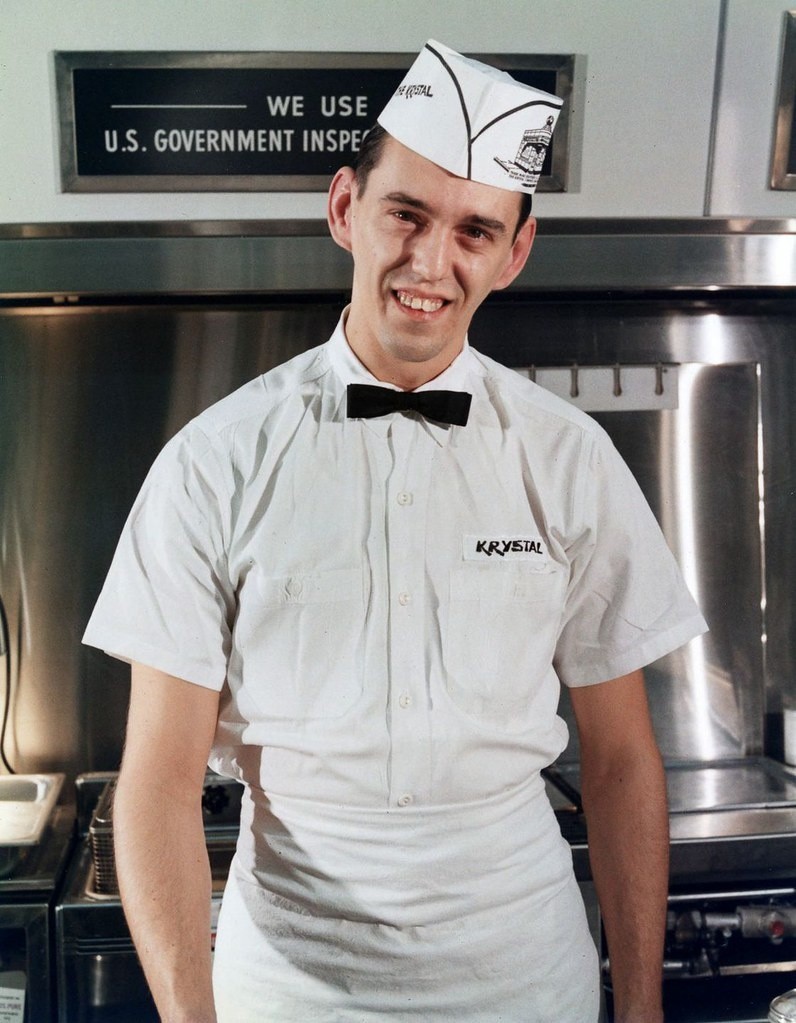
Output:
[346,384,474,427]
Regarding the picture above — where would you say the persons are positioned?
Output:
[83,40,709,1023]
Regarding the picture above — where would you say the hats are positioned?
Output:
[375,37,566,194]
